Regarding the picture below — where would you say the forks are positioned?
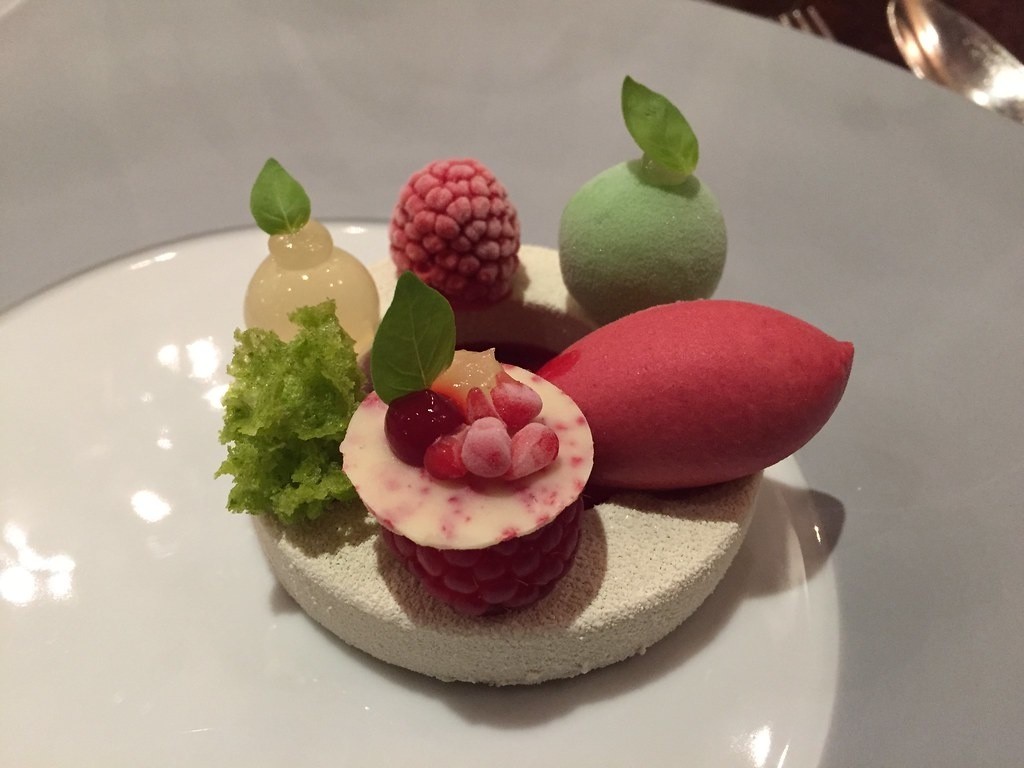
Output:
[763,6,837,43]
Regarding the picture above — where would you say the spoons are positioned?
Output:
[886,0,1024,126]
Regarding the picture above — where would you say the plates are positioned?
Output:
[0,0,1024,768]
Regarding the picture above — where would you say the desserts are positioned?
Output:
[211,74,855,690]
